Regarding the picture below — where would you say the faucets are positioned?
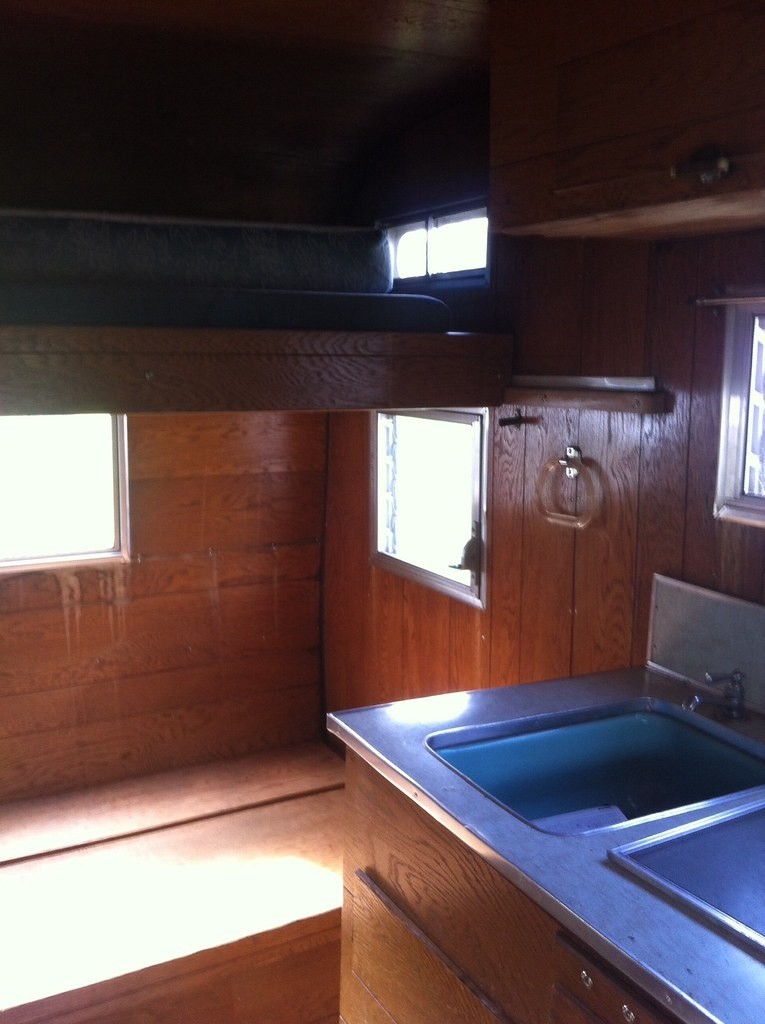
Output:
[681,669,745,723]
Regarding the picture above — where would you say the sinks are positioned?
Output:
[607,798,765,964]
[427,697,764,836]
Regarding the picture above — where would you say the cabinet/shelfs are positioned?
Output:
[339,744,679,1024]
